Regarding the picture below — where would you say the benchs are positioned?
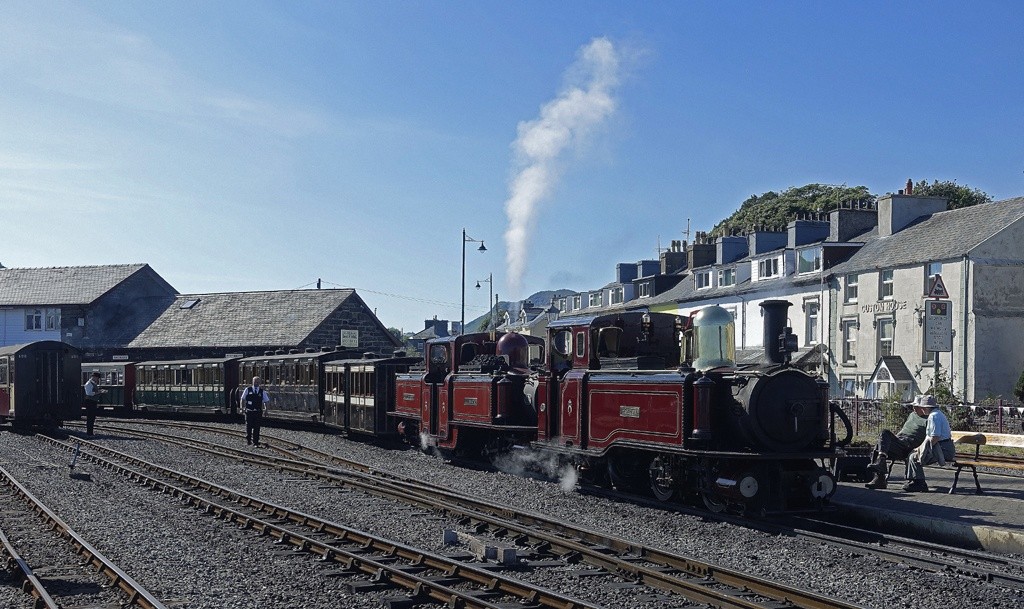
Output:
[886,433,986,495]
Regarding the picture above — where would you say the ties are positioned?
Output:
[255,387,257,393]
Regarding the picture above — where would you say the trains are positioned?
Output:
[0,340,84,432]
[79,311,854,521]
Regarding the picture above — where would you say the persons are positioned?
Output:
[239,376,270,448]
[864,395,929,489]
[83,372,103,437]
[902,394,955,493]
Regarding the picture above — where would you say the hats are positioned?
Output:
[907,395,926,406]
[90,372,101,377]
[918,395,939,408]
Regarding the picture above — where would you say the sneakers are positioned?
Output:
[903,479,928,492]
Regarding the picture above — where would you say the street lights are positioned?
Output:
[462,226,488,335]
[476,273,493,317]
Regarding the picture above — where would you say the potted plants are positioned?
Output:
[835,436,872,482]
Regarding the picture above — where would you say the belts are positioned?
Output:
[938,438,952,443]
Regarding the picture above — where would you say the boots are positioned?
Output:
[865,474,887,488]
[867,456,887,473]
